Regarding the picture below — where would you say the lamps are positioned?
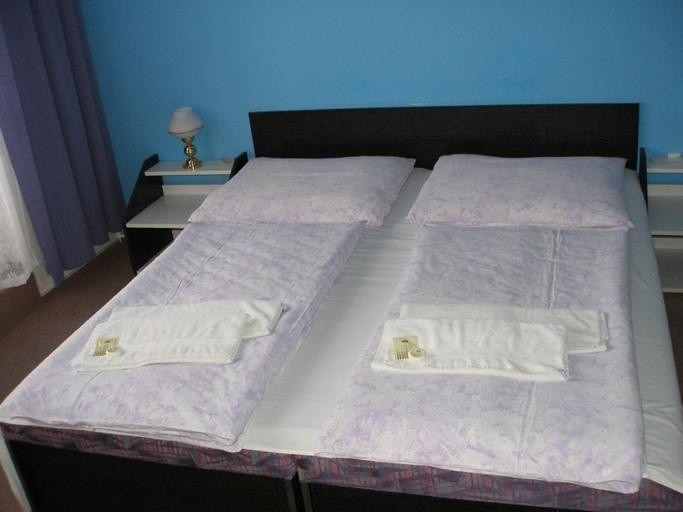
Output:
[167,107,205,171]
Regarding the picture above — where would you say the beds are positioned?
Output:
[0,102,683,512]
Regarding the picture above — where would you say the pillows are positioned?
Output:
[186,153,635,232]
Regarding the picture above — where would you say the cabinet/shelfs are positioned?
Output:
[121,151,248,276]
[638,147,683,294]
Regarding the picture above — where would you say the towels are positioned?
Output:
[68,299,290,372]
[370,301,612,385]
